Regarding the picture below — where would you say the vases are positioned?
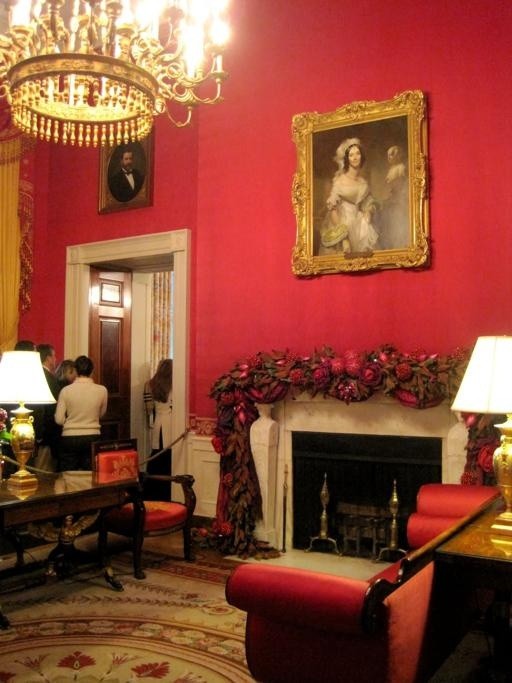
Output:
[0,459,5,485]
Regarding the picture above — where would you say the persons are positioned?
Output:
[321,137,378,256]
[109,151,143,201]
[56,360,76,386]
[8,341,43,446]
[34,345,59,453]
[56,356,108,470]
[144,360,172,450]
[375,147,411,251]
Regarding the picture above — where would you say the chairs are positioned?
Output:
[91,438,196,580]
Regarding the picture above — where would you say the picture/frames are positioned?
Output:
[99,127,155,214]
[290,90,431,277]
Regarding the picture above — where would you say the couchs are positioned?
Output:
[225,484,504,683]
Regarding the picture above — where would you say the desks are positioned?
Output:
[435,505,512,683]
[0,471,138,630]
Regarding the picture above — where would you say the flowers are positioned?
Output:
[0,408,8,456]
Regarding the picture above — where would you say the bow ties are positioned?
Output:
[124,169,131,172]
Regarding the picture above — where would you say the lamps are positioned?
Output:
[0,350,58,487]
[451,336,512,535]
[0,0,231,148]
